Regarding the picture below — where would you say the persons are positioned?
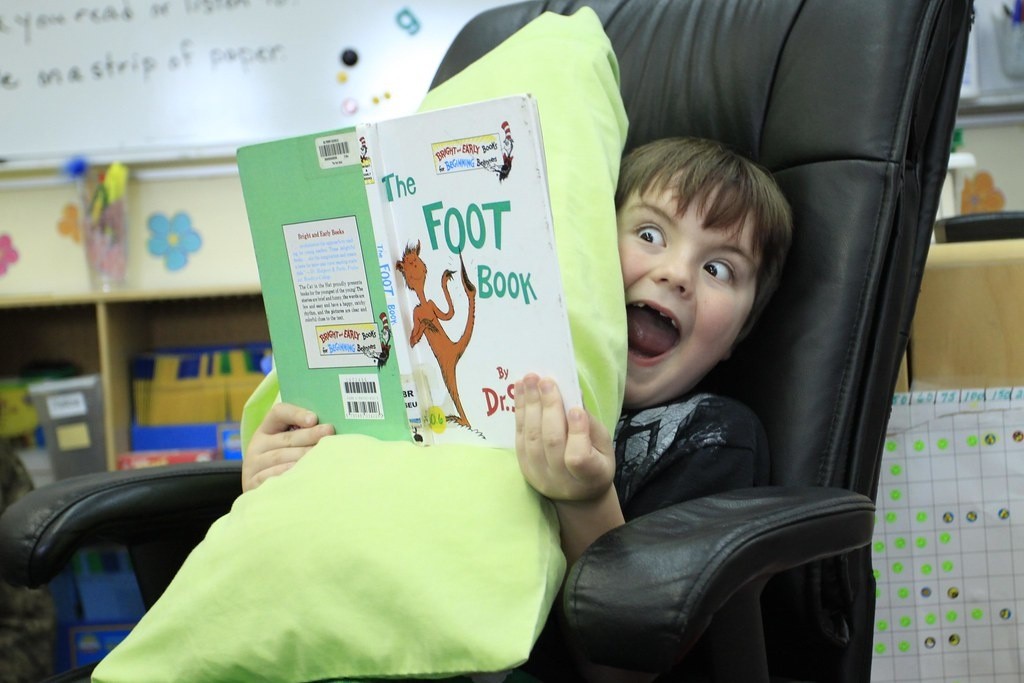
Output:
[241,134,794,683]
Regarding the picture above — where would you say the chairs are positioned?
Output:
[0,0,973,683]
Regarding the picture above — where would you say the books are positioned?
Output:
[238,94,583,449]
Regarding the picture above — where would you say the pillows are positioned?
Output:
[91,5,625,683]
